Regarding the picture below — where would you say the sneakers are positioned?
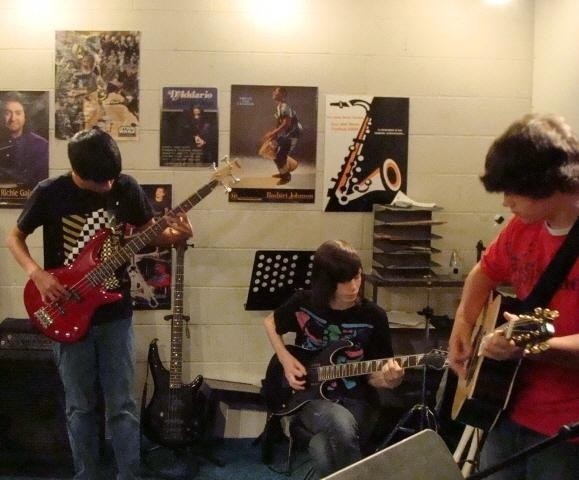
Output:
[272,170,291,185]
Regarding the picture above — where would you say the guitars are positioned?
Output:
[143,242,204,450]
[451,287,561,430]
[23,154,241,344]
[263,340,450,416]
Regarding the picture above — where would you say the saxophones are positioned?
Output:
[330,98,403,206]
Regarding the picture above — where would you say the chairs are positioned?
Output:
[319,429,466,480]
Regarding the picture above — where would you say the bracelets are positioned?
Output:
[168,227,181,239]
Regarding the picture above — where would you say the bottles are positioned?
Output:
[447,250,463,281]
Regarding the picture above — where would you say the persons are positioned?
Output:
[6,130,194,480]
[262,238,409,479]
[0,91,49,189]
[443,112,579,480]
[186,104,214,164]
[265,87,301,186]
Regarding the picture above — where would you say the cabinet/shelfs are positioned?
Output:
[360,206,470,346]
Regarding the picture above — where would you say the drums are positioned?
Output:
[259,139,300,172]
